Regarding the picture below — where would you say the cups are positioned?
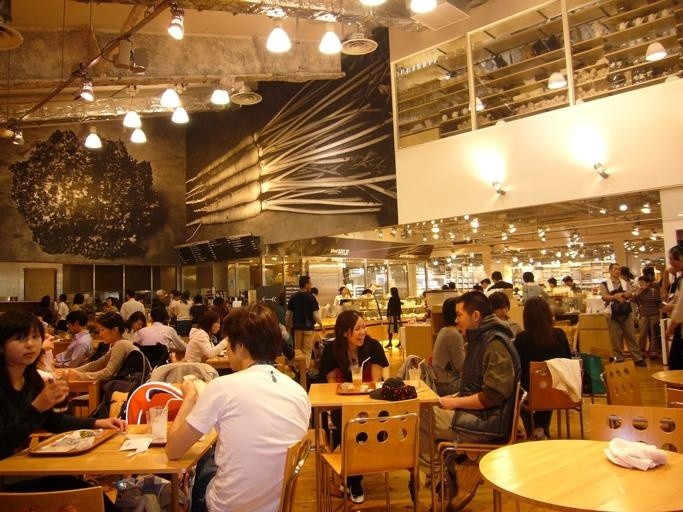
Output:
[148,406,168,442]
[408,367,422,388]
[50,374,71,413]
[350,365,364,389]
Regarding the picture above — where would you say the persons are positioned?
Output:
[0,308,131,512]
[166,302,312,512]
[333,287,403,348]
[27,277,331,389]
[409,243,682,511]
[319,310,390,504]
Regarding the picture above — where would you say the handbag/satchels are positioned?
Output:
[577,352,606,394]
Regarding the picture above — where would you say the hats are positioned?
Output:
[368,378,406,400]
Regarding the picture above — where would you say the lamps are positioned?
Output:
[469,96,484,113]
[646,42,666,60]
[547,72,567,89]
[0,21,25,147]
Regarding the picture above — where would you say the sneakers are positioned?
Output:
[350,485,364,502]
[339,480,350,494]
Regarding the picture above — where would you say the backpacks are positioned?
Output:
[608,287,632,324]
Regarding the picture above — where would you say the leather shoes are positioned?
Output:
[634,359,646,366]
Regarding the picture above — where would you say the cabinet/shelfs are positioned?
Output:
[340,298,427,326]
[391,1,683,151]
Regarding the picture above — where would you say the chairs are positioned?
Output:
[602,362,646,406]
[590,402,683,453]
[439,384,520,511]
[517,359,585,439]
[318,402,420,512]
[280,437,308,512]
[402,305,617,374]
[0,359,214,512]
[666,388,683,405]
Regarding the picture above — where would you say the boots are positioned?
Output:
[451,456,484,511]
